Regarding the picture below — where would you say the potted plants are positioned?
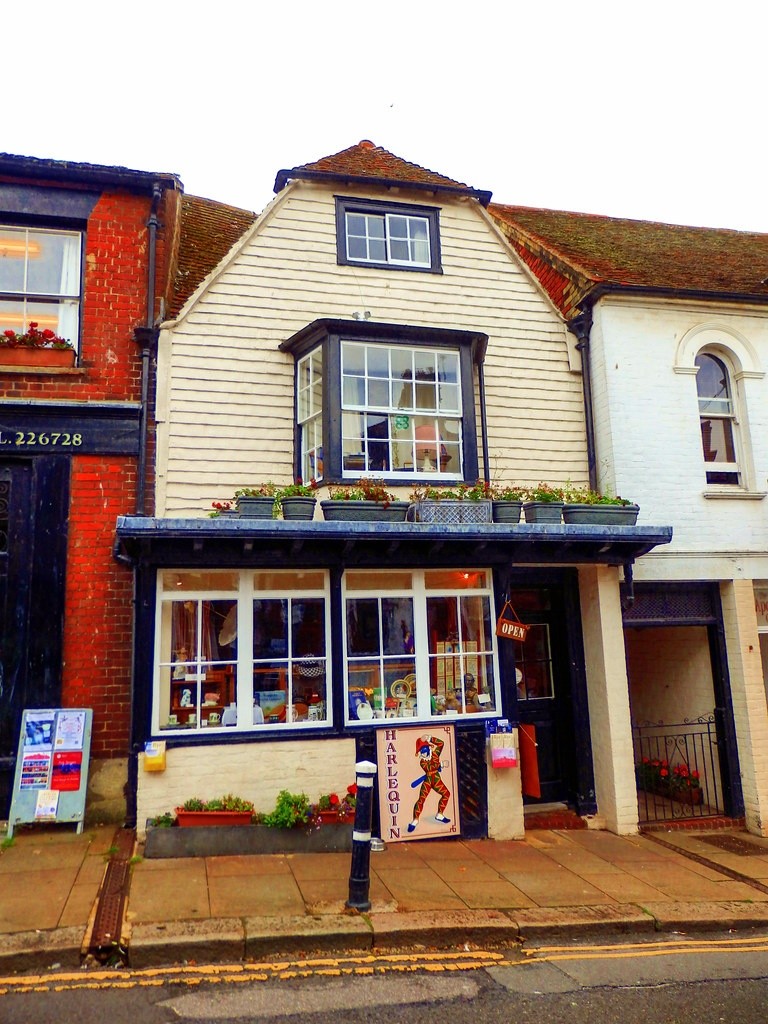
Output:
[320,474,410,521]
[562,477,641,526]
[175,795,256,826]
[407,479,494,523]
[491,483,524,523]
[280,478,318,520]
[521,480,564,525]
[0,347,77,366]
[235,483,282,519]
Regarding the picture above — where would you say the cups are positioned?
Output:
[285,705,298,722]
[308,705,322,721]
[188,714,196,722]
[209,713,220,721]
[168,715,177,723]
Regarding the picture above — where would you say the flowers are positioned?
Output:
[265,783,360,836]
[0,320,72,349]
[211,500,234,510]
[638,756,701,792]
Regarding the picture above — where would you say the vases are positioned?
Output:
[311,810,357,825]
[217,510,238,520]
[644,782,700,804]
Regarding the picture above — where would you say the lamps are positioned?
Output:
[410,424,441,473]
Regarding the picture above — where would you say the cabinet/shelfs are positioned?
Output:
[170,677,226,721]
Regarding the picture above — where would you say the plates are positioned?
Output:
[186,722,197,725]
[166,722,180,725]
[207,721,220,724]
